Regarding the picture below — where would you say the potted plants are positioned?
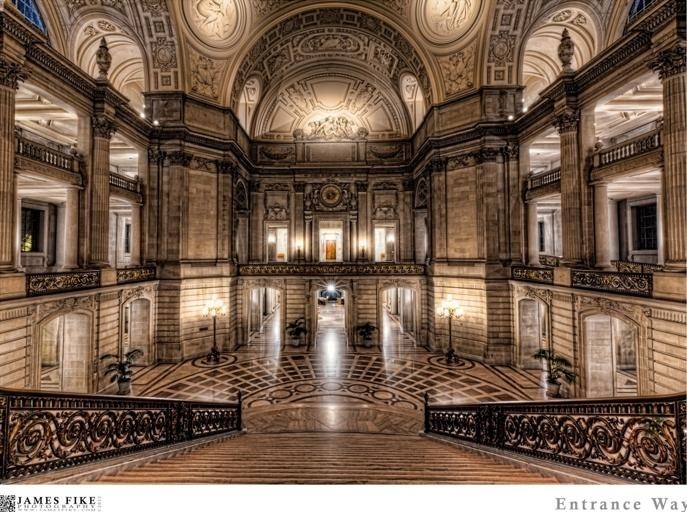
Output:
[531,348,576,397]
[98,348,143,396]
[285,317,307,347]
[354,320,380,347]
